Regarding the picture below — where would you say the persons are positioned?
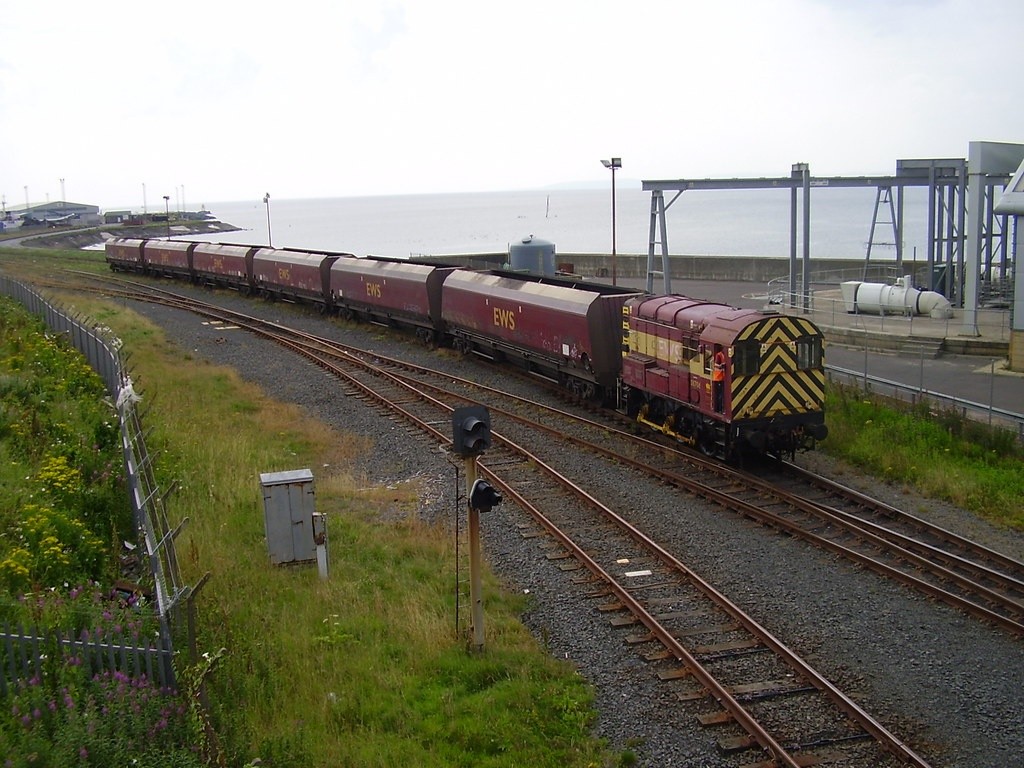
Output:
[712,352,724,414]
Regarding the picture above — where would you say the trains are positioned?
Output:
[105,235,831,467]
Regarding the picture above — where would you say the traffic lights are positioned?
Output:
[452,405,493,455]
[469,479,503,513]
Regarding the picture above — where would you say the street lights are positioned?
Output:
[599,157,624,285]
[163,196,173,241]
[262,193,273,247]
